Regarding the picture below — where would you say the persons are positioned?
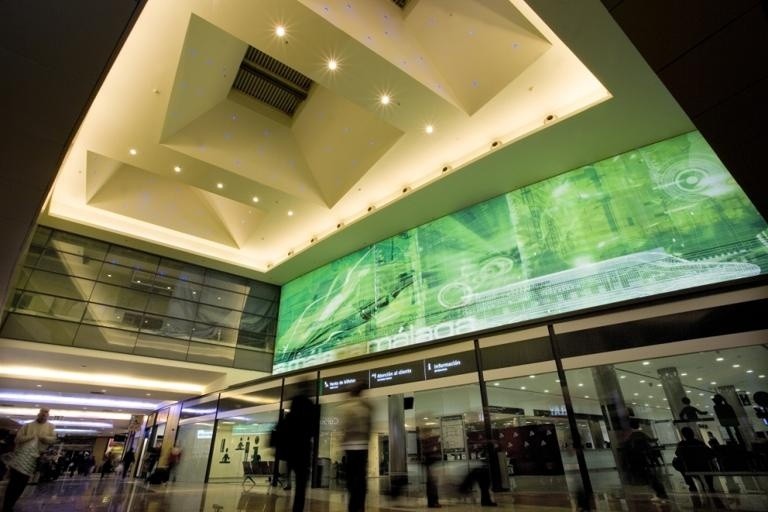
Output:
[1,404,57,511]
[706,430,720,448]
[622,416,673,505]
[383,468,442,508]
[671,426,716,493]
[0,429,185,491]
[283,460,294,490]
[473,468,499,507]
[334,378,376,512]
[281,378,320,511]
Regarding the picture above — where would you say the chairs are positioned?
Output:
[241,461,283,486]
[677,443,768,494]
